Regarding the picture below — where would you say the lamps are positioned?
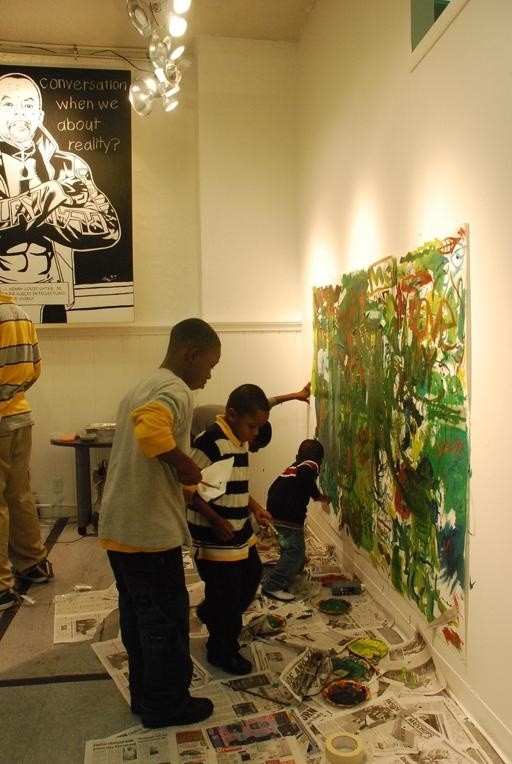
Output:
[126,0,193,115]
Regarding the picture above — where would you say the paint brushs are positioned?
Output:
[201,481,222,491]
[266,522,299,550]
[220,682,290,708]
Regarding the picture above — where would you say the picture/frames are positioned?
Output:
[409,0,470,73]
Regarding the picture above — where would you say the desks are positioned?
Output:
[51,437,116,539]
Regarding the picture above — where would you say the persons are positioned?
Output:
[260,439,332,601]
[1,73,121,311]
[98,318,221,730]
[0,292,53,608]
[181,383,272,673]
[190,381,312,449]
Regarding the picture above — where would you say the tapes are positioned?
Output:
[324,731,366,764]
